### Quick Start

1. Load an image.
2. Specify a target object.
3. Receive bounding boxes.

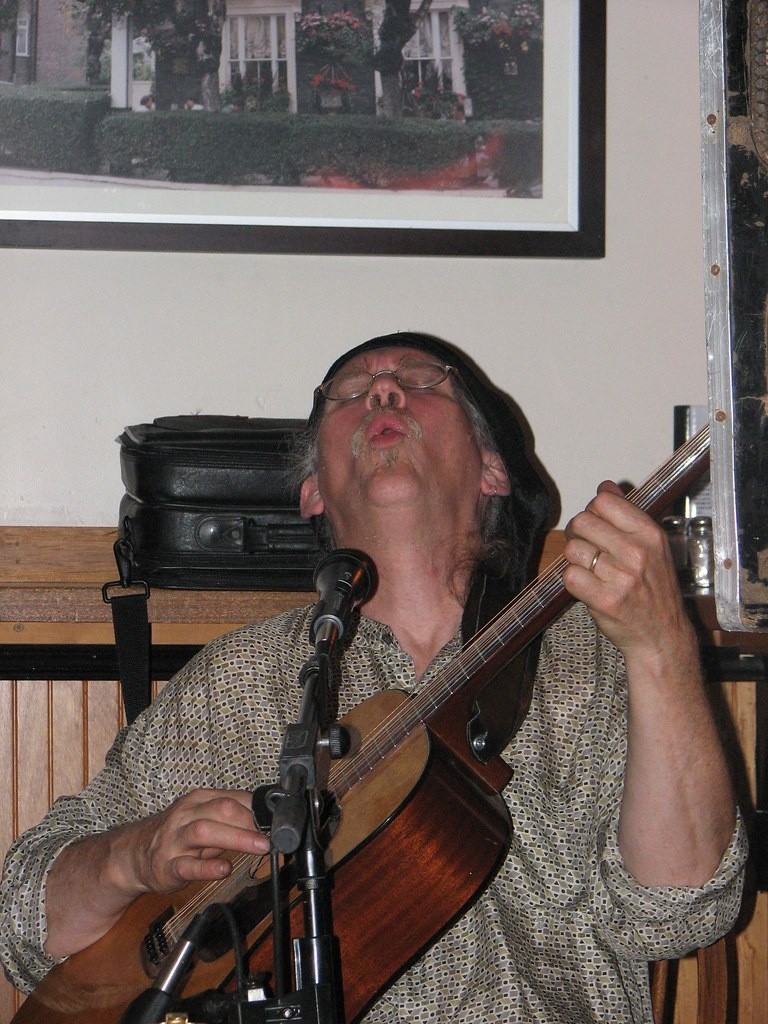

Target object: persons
[0,335,750,1024]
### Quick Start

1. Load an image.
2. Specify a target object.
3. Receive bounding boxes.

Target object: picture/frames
[1,0,608,261]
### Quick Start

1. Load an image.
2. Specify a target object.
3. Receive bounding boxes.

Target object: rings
[589,551,600,573]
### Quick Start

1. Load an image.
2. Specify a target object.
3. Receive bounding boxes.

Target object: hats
[306,332,548,594]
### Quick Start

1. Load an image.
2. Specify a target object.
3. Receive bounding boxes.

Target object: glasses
[311,362,486,459]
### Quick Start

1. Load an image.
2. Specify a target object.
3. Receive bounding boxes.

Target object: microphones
[312,547,379,663]
[119,912,211,1024]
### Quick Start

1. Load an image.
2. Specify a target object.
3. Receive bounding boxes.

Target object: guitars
[9,418,714,1024]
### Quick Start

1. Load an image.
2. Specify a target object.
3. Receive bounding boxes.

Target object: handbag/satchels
[102,415,313,725]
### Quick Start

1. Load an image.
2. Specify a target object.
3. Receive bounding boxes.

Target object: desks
[1,526,768,1024]
